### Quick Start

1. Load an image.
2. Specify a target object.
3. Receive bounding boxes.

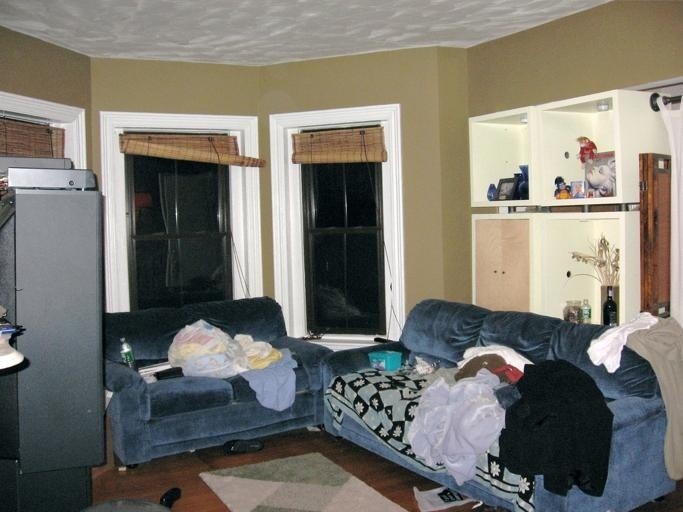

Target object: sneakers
[222,436,264,454]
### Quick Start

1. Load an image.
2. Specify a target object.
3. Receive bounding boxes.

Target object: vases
[601,286,617,326]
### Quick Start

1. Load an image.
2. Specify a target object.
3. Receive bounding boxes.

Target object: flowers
[569,234,619,287]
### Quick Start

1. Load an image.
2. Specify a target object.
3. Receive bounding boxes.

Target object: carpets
[198,452,409,512]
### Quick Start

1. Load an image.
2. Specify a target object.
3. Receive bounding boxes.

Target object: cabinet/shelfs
[474,218,529,314]
[532,215,623,326]
[468,91,621,204]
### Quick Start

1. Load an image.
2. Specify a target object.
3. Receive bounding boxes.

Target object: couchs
[326,298,678,510]
[101,298,332,469]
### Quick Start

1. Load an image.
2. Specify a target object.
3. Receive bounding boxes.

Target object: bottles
[604,286,617,327]
[120,337,136,371]
[563,299,591,325]
